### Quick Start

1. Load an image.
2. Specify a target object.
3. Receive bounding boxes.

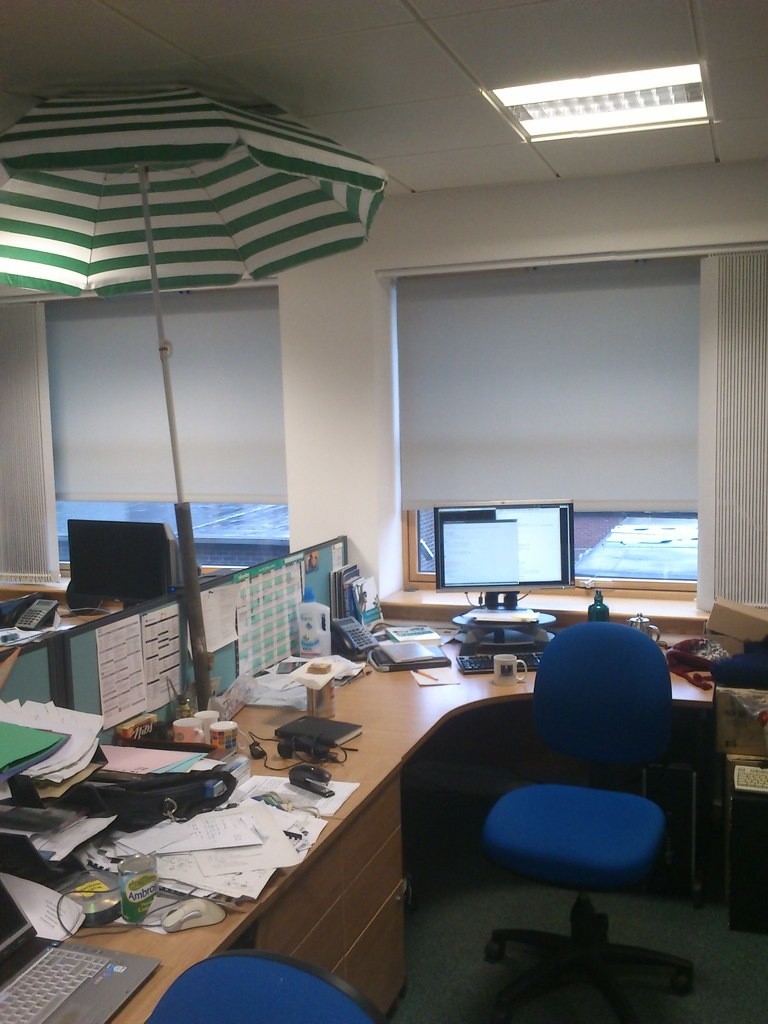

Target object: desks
[0,535,718,1024]
[724,754,768,932]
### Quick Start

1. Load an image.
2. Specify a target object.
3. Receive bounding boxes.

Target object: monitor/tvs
[67,518,183,602]
[433,499,576,594]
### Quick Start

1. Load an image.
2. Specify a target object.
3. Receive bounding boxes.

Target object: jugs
[627,613,661,643]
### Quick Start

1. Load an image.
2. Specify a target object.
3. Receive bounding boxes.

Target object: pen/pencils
[351,671,373,682]
[340,676,354,687]
[413,669,439,682]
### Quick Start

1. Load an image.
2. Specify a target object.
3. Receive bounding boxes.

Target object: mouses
[162,898,226,933]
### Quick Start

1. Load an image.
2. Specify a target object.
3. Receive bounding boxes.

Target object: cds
[53,870,121,927]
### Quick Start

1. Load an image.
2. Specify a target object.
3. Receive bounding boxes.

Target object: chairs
[481,622,695,1024]
[145,950,390,1024]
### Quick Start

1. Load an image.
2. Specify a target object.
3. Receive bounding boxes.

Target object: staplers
[288,765,336,798]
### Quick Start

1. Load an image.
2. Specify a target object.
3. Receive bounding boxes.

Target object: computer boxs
[642,764,696,895]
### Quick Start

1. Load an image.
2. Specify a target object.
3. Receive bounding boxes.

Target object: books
[330,564,383,631]
[385,625,441,646]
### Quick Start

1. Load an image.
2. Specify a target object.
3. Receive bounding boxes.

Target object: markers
[2,633,20,644]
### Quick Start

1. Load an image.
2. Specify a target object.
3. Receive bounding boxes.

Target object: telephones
[3,595,59,631]
[331,617,379,655]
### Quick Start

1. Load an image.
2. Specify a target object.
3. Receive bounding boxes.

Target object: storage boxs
[715,681,768,756]
[116,714,156,747]
[705,596,768,642]
[703,622,744,656]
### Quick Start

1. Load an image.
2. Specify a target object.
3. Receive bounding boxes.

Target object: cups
[209,720,250,755]
[193,710,220,745]
[172,716,207,744]
[493,654,528,686]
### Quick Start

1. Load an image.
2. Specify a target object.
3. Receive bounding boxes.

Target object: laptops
[0,880,162,1024]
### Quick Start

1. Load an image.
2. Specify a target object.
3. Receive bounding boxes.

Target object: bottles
[298,587,331,659]
[588,589,610,622]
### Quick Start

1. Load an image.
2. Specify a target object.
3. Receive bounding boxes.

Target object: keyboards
[456,652,542,675]
